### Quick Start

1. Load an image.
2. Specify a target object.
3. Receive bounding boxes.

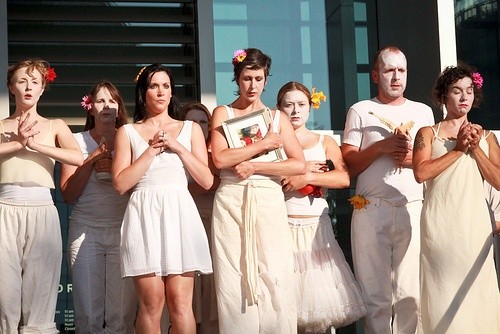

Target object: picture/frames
[221,107,284,163]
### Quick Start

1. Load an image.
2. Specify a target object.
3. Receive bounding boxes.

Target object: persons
[60,62,222,334]
[276,81,366,334]
[413,65,500,334]
[0,59,83,334]
[342,47,435,334]
[212,49,306,334]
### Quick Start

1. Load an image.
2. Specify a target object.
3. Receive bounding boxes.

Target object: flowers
[81,95,94,111]
[348,196,370,210]
[310,86,327,109]
[232,49,248,64]
[44,67,57,83]
[471,73,484,90]
